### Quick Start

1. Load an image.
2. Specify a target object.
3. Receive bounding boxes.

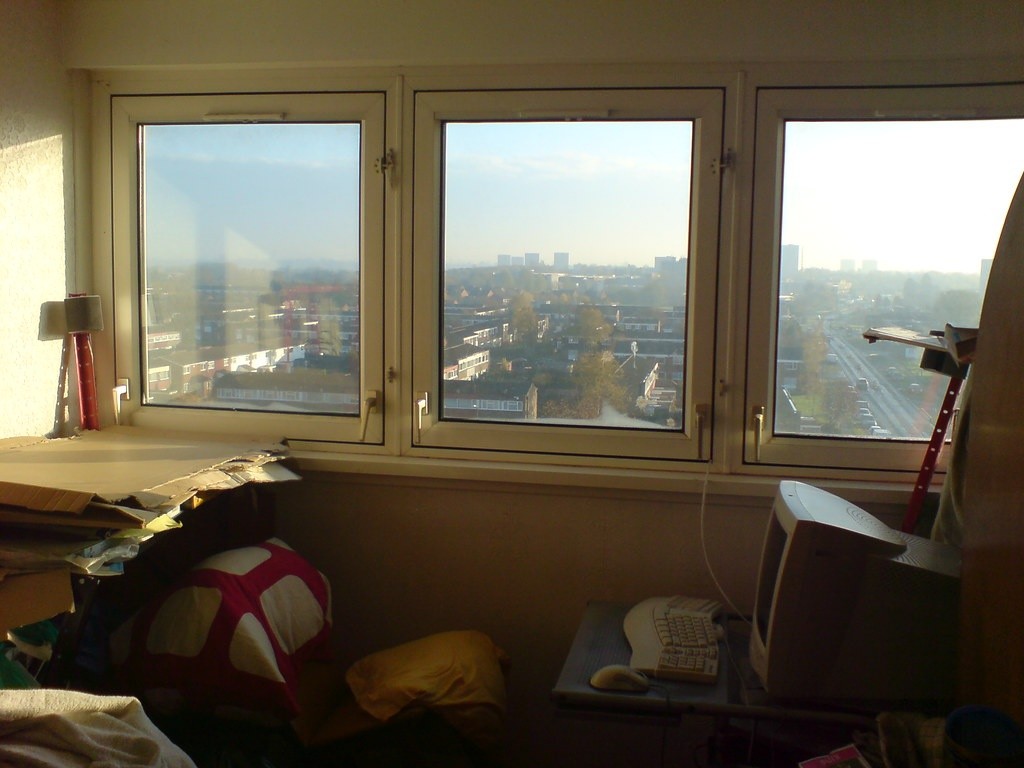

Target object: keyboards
[623,595,727,684]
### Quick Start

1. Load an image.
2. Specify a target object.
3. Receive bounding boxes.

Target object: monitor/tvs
[749,479,964,704]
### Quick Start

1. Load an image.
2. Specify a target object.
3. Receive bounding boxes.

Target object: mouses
[589,664,650,691]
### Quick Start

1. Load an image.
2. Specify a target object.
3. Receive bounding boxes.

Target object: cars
[855,400,869,409]
[868,425,881,434]
[861,413,875,420]
[873,429,890,438]
[860,407,871,414]
[856,378,869,390]
[863,420,877,427]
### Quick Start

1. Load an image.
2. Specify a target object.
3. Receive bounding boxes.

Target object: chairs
[201,537,510,768]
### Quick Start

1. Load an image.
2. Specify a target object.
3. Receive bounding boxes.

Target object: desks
[551,600,880,768]
